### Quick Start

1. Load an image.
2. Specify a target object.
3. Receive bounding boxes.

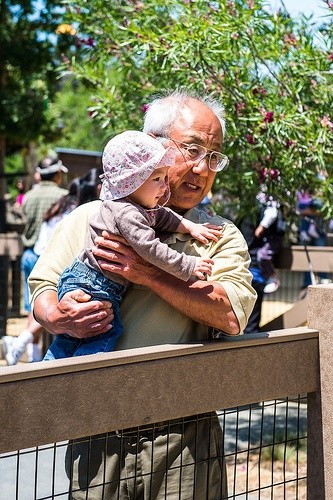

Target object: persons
[64,89,258,500]
[42,129,223,365]
[237,173,329,333]
[0,153,102,366]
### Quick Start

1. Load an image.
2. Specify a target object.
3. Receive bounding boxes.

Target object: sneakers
[0,336,24,366]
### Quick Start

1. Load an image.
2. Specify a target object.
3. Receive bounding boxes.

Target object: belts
[91,415,196,438]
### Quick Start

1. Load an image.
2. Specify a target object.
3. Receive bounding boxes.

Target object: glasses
[158,136,229,172]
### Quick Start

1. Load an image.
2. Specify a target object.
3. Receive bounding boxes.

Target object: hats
[35,160,68,174]
[100,130,171,200]
